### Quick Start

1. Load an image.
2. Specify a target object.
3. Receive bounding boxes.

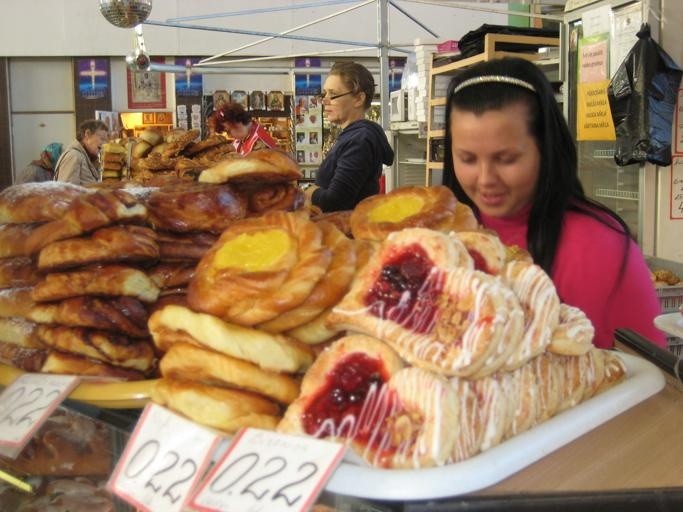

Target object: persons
[215,95,226,110]
[311,151,320,163]
[297,98,307,115]
[442,58,669,360]
[54,119,110,185]
[297,133,305,145]
[310,133,318,144]
[301,59,394,214]
[17,143,62,184]
[213,104,275,156]
[270,94,282,108]
[141,71,153,91]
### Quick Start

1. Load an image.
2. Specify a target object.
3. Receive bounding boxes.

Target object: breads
[135,130,240,188]
[149,184,478,433]
[649,269,683,288]
[280,228,623,468]
[2,150,304,382]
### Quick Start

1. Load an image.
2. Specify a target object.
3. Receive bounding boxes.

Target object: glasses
[317,85,378,106]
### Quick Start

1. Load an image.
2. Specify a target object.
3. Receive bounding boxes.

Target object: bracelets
[302,183,312,192]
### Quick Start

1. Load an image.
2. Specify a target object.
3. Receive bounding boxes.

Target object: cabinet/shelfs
[0,378,681,512]
[424,33,562,190]
[200,83,295,156]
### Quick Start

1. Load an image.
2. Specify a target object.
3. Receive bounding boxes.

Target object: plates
[652,308,682,341]
